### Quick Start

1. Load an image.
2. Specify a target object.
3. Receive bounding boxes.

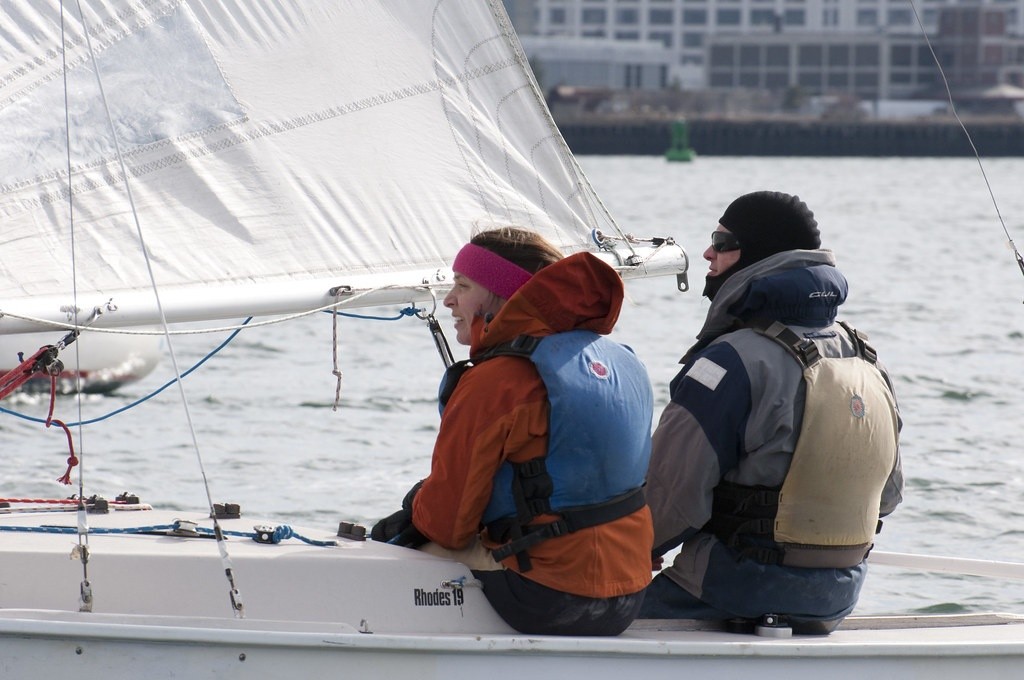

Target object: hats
[718,191,821,260]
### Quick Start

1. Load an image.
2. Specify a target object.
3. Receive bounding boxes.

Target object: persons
[636,190,903,637]
[372,227,654,637]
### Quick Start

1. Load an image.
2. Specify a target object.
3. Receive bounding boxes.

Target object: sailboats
[0,0,1024,679]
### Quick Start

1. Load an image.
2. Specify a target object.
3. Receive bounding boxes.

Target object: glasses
[711,231,739,253]
